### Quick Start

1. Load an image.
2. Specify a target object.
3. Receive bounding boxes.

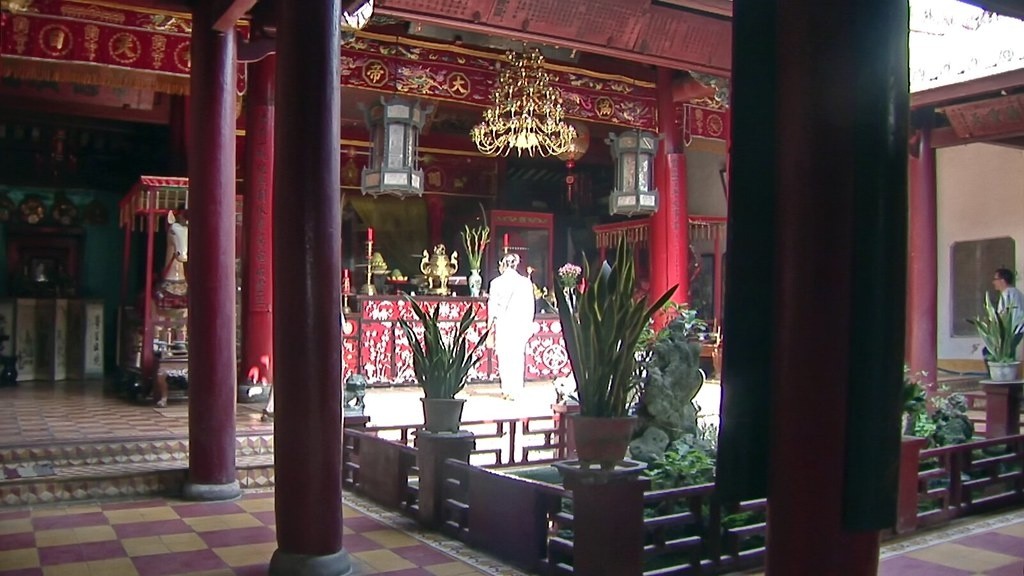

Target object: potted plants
[398,289,490,436]
[551,230,681,470]
[966,287,1024,382]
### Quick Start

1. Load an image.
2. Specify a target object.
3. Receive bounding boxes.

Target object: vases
[469,269,481,297]
[563,285,576,316]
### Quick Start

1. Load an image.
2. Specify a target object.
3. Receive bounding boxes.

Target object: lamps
[469,42,580,156]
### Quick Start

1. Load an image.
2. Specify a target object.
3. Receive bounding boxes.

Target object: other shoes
[500,393,515,400]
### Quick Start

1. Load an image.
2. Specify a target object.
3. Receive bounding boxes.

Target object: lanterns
[604,129,665,218]
[360,94,434,199]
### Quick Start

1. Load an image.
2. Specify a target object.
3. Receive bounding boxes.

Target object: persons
[992,269,1024,360]
[487,253,535,400]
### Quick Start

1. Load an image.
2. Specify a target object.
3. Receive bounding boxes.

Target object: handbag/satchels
[486,323,496,349]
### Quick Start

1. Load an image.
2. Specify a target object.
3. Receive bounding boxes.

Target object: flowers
[557,263,580,286]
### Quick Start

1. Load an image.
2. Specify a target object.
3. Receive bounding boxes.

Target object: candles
[369,227,372,241]
[345,269,348,279]
[504,233,508,247]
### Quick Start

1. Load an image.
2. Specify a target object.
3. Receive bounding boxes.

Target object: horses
[151,209,188,354]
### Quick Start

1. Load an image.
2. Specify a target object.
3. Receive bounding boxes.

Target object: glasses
[992,277,1002,281]
